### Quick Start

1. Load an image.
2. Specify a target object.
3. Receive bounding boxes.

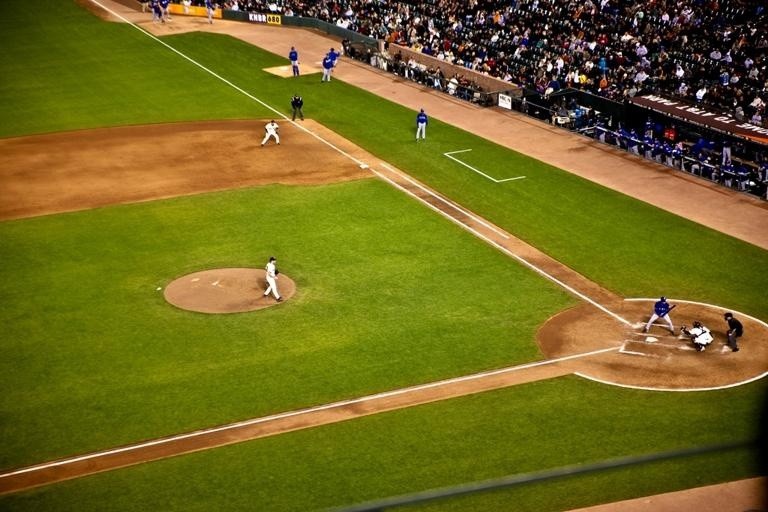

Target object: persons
[644,296,676,337]
[262,256,283,302]
[724,313,744,353]
[416,109,428,141]
[150,0,319,18]
[289,47,300,76]
[290,94,304,120]
[319,0,526,112]
[680,319,714,352]
[261,120,280,146]
[208,6,213,22]
[152,3,170,21]
[526,0,768,199]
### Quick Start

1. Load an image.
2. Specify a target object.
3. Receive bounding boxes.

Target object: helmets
[692,321,700,328]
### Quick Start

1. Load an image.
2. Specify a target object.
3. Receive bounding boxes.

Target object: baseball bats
[662,305,675,317]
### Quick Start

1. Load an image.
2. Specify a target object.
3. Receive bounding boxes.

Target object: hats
[724,313,732,317]
[421,109,424,112]
[661,297,666,302]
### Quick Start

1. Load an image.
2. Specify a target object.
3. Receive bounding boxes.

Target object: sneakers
[732,347,737,352]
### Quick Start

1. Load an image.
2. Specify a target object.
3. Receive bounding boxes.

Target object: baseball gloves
[680,326,686,333]
